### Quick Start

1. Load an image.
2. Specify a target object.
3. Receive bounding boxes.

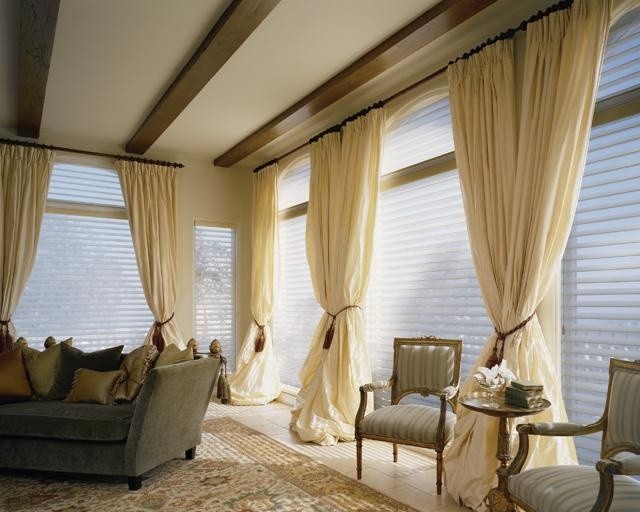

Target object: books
[504,380,543,409]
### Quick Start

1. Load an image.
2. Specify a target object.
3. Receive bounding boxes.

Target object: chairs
[502,358,640,512]
[353,335,463,495]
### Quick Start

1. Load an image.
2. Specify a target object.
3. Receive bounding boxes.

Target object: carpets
[1,416,423,512]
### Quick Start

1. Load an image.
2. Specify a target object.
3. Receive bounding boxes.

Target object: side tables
[456,390,552,512]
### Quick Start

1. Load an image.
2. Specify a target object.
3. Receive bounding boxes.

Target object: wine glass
[477,377,506,410]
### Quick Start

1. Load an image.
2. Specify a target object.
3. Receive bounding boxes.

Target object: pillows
[0,336,195,407]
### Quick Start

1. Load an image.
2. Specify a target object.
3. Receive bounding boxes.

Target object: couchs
[0,335,222,490]
